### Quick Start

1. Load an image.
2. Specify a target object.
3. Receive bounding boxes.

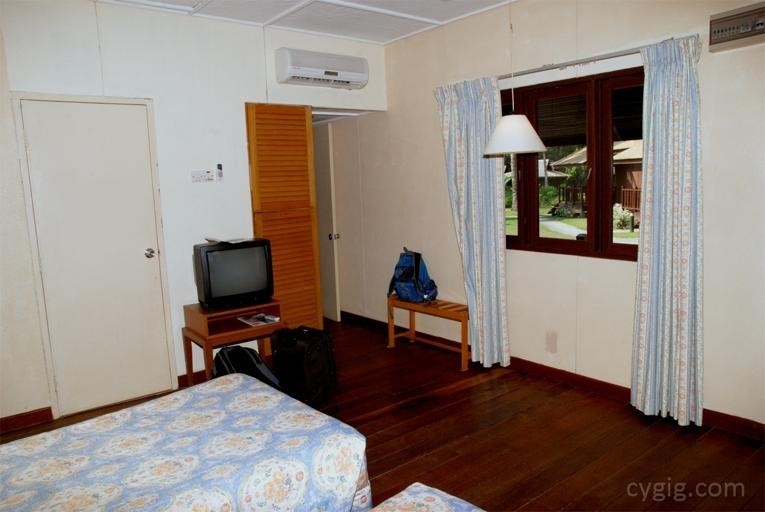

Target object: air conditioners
[273,45,373,91]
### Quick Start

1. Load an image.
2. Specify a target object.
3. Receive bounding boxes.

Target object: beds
[0,373,487,510]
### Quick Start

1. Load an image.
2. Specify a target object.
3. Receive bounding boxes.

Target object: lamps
[480,0,551,159]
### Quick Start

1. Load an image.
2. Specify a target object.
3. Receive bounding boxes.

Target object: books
[237,314,281,328]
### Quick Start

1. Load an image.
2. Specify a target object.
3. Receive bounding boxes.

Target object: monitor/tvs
[193,237,274,312]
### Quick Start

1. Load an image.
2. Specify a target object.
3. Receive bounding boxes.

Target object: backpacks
[387,247,437,303]
[211,346,284,392]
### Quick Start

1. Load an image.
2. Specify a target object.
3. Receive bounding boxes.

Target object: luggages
[271,325,336,404]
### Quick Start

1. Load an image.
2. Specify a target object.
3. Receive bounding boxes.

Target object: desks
[387,292,483,372]
[181,300,289,385]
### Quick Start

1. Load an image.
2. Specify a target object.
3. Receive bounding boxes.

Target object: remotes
[266,315,280,322]
